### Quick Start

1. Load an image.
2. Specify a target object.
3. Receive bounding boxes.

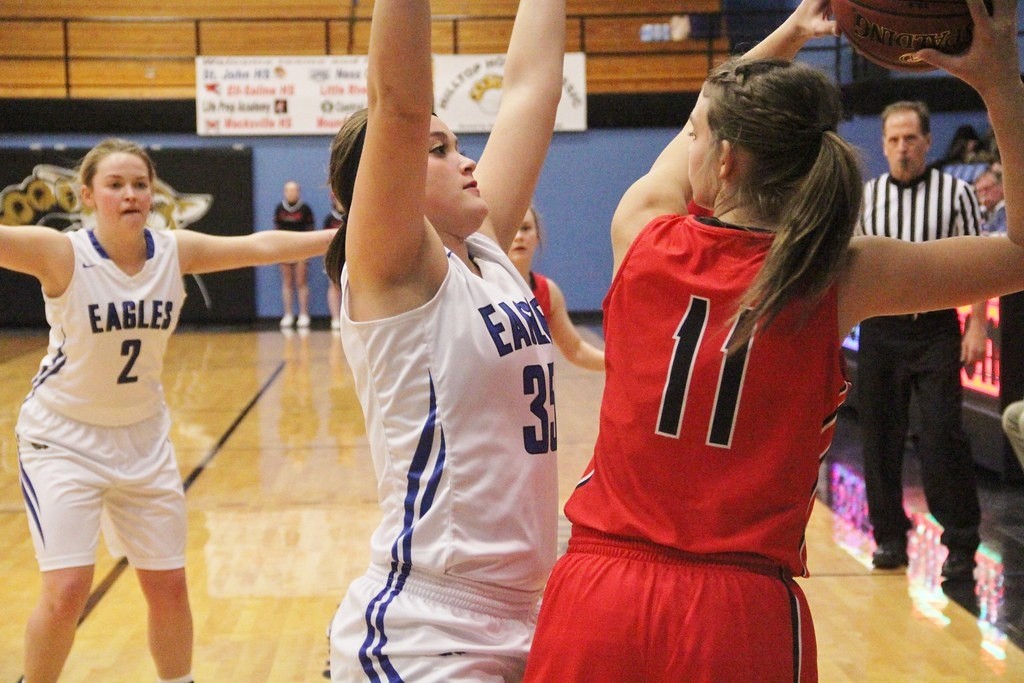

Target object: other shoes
[941,553,977,577]
[279,315,295,327]
[296,315,310,327]
[331,318,340,330]
[872,537,908,567]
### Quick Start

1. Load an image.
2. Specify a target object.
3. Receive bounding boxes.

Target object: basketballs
[830,0,993,73]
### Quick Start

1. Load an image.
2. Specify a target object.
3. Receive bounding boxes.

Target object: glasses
[973,181,1000,196]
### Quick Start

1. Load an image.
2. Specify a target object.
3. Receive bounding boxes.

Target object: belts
[884,309,952,324]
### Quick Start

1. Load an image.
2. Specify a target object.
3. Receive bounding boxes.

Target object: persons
[852,89,1024,584]
[520,0,1024,683]
[0,140,337,683]
[270,0,606,683]
